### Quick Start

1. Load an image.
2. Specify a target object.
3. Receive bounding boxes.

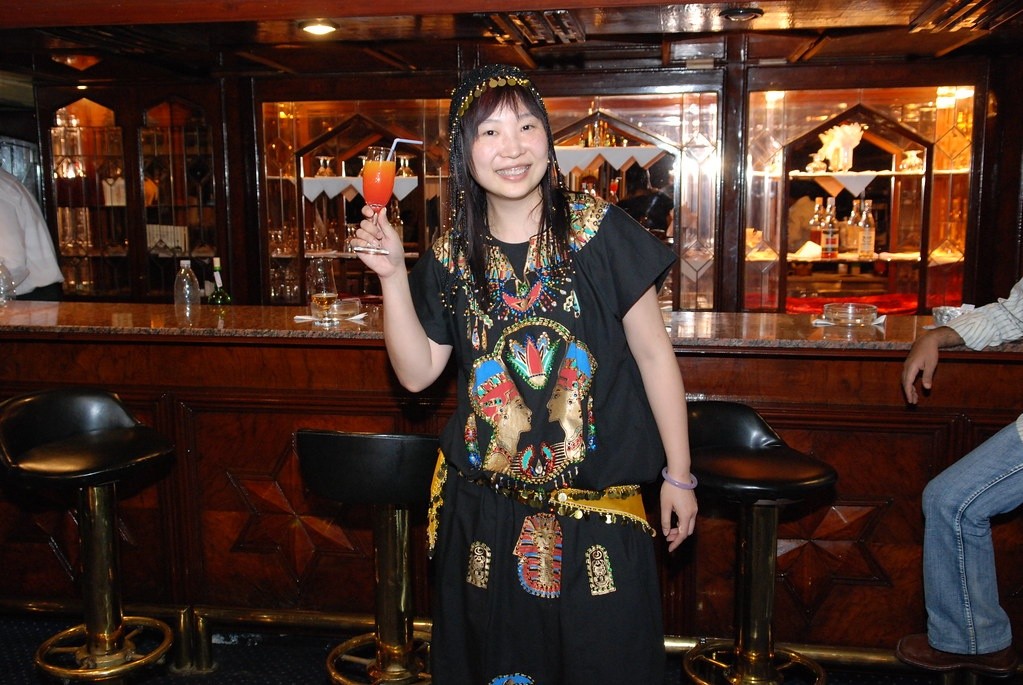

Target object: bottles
[579,105,616,147]
[809,196,825,246]
[208,257,231,306]
[846,199,861,253]
[821,198,839,257]
[390,199,404,246]
[858,200,875,257]
[174,260,201,325]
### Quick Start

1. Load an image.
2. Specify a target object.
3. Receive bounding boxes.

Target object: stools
[686,400,839,685]
[294,428,443,685]
[0,386,179,681]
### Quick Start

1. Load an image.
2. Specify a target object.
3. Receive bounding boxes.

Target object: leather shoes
[897,633,1014,677]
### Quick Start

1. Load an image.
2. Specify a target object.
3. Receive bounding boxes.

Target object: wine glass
[316,154,415,177]
[310,258,338,327]
[901,150,923,171]
[269,222,360,254]
[352,144,396,254]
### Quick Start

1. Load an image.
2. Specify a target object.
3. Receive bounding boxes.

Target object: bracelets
[661,467,697,490]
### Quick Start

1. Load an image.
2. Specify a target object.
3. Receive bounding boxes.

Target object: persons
[353,71,699,685]
[612,163,676,297]
[894,277,1023,676]
[1,166,68,301]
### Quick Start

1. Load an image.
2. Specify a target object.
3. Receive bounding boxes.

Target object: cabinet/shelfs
[43,122,962,318]
